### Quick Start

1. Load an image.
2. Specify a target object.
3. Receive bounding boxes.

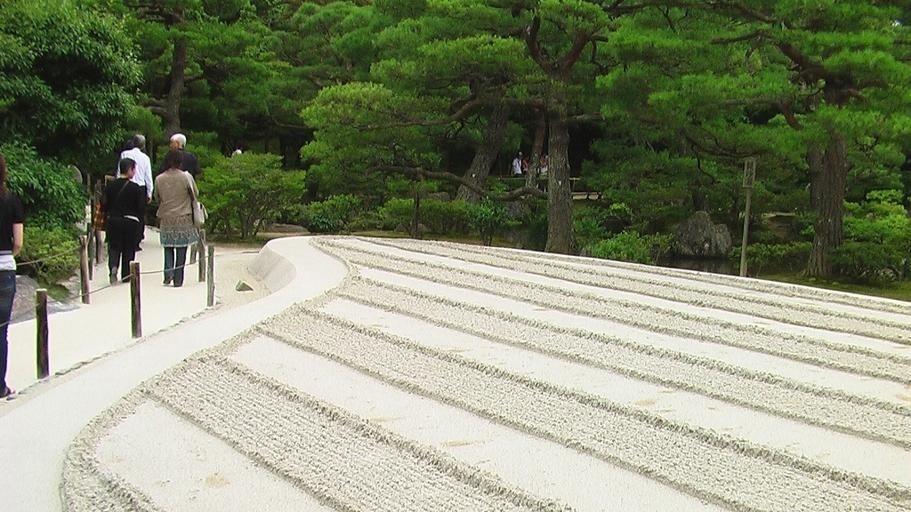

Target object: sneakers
[109,263,186,287]
[1,385,16,398]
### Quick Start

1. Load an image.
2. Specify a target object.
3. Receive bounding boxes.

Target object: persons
[0,152,27,399]
[115,133,156,253]
[509,150,549,176]
[232,142,244,156]
[158,132,199,182]
[154,151,200,289]
[98,156,148,285]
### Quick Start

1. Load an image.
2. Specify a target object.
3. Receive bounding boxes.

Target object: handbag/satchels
[185,168,209,225]
